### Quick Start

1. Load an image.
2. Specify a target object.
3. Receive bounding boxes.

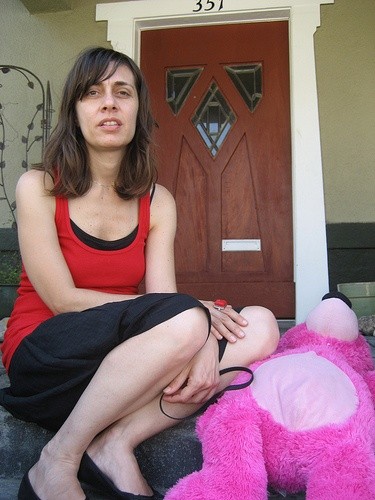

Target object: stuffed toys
[164,293,375,500]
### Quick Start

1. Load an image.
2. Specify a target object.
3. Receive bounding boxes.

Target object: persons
[0,45,279,500]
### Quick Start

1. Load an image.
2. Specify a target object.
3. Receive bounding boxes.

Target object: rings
[213,299,228,311]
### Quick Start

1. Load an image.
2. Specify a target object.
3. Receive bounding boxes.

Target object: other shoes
[18,467,89,500]
[78,450,165,500]
